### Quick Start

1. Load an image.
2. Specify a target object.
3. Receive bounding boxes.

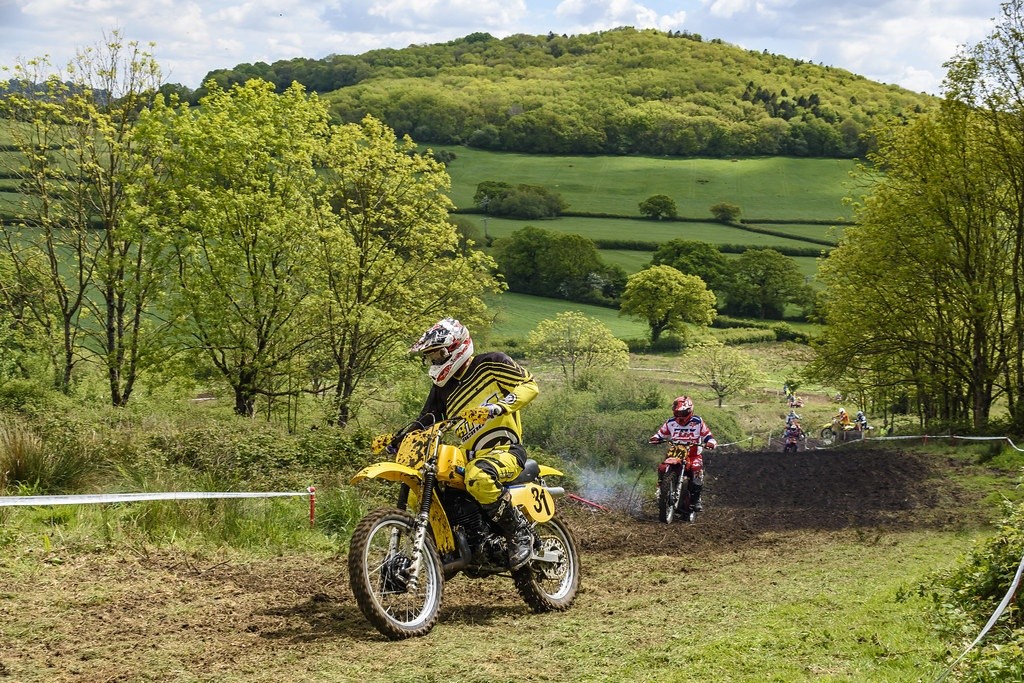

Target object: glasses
[422,325,469,367]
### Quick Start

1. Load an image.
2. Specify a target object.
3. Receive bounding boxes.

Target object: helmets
[671,396,694,426]
[790,410,795,416]
[856,411,864,418]
[790,424,798,433]
[409,316,474,387]
[839,408,846,415]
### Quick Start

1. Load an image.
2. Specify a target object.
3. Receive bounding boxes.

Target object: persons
[782,410,805,445]
[650,395,717,513]
[384,315,542,571]
[835,408,851,431]
[854,411,867,430]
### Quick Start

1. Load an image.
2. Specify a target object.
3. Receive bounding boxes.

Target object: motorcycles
[657,438,712,524]
[784,417,874,457]
[347,405,582,640]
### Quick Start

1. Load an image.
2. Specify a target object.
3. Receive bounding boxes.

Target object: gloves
[480,402,506,418]
[650,438,659,444]
[385,436,400,455]
[705,442,714,450]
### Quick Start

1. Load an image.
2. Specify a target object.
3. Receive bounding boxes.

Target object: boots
[690,482,702,510]
[488,495,535,571]
